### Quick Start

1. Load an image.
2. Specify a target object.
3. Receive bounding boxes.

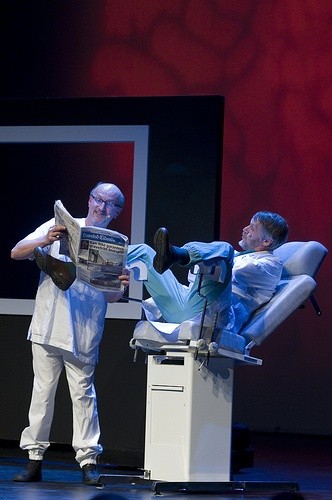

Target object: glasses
[92,195,120,208]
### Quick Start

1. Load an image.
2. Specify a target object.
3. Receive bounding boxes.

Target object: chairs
[124,242,328,366]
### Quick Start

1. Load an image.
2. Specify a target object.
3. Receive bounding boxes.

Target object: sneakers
[13,461,42,481]
[82,463,99,484]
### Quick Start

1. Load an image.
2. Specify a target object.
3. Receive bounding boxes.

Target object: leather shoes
[33,247,76,291]
[153,227,173,274]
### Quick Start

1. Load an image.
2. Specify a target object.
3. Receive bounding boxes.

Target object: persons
[33,211,289,335]
[11,183,130,487]
